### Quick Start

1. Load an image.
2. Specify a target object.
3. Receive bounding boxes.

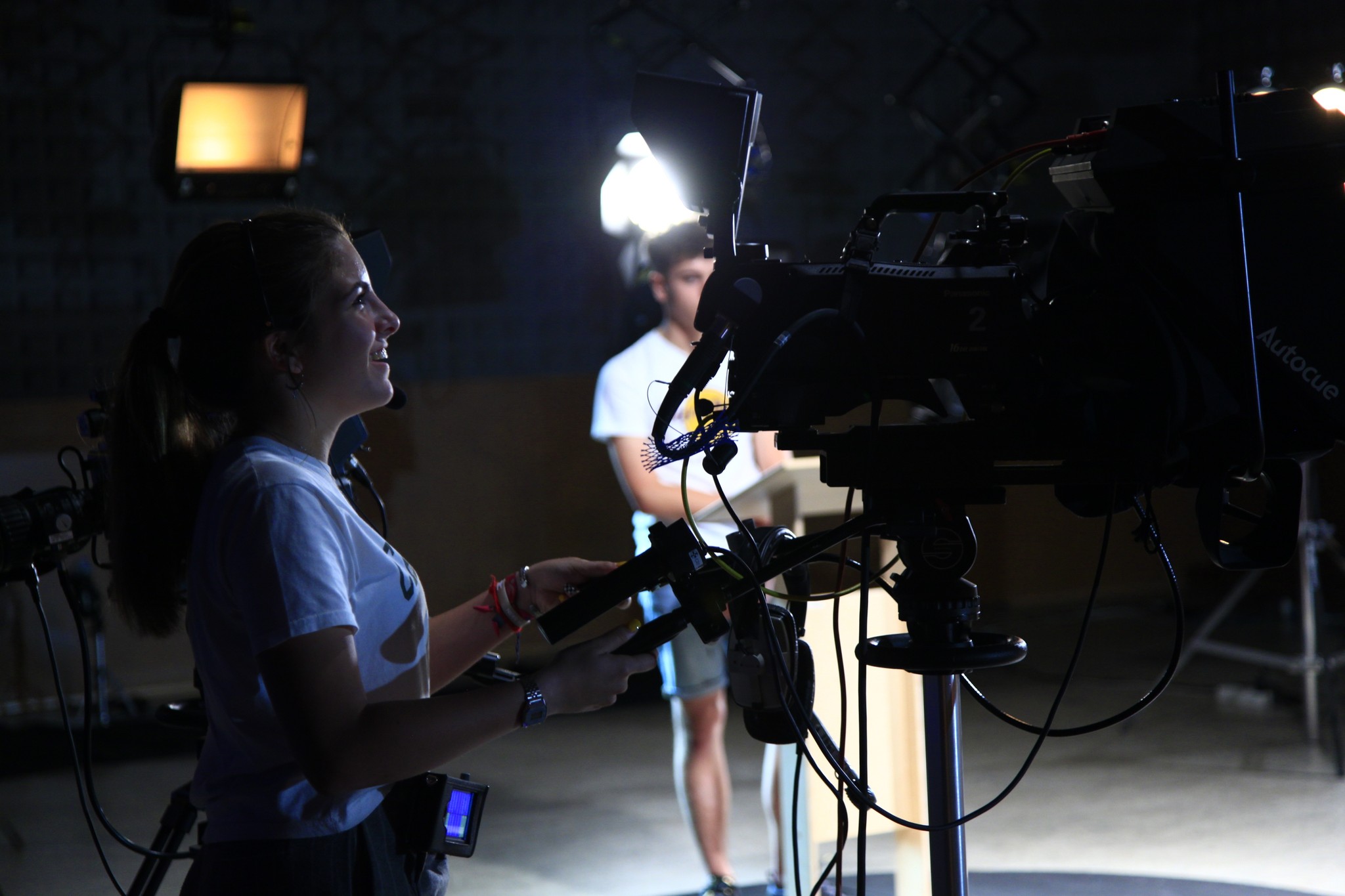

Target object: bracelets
[472,565,543,638]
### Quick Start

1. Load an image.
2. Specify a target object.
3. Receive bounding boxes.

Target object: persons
[96,208,659,896]
[591,222,811,894]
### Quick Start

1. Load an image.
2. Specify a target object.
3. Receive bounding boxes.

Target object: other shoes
[701,877,736,896]
[766,878,846,896]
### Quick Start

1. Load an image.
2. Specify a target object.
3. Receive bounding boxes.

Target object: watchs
[516,675,547,727]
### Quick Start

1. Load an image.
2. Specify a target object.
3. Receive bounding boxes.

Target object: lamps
[164,67,315,207]
